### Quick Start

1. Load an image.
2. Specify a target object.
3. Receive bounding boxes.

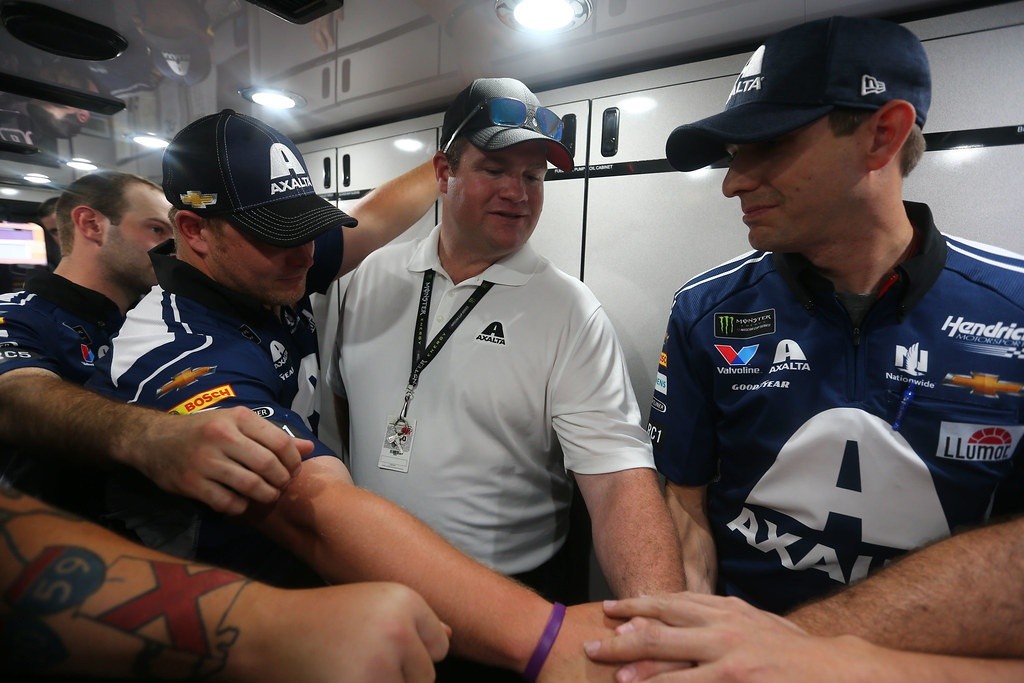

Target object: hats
[162,108,359,249]
[664,16,933,173]
[440,77,574,173]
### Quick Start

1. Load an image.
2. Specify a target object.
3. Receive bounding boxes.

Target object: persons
[314,77,687,600]
[583,590,1024,682]
[110,107,634,683]
[1,0,345,140]
[0,172,317,525]
[645,15,1023,656]
[38,198,63,245]
[0,480,455,683]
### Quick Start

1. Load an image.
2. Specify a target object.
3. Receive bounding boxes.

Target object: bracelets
[521,601,567,683]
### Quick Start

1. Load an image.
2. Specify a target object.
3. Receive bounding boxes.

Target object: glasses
[442,97,563,153]
